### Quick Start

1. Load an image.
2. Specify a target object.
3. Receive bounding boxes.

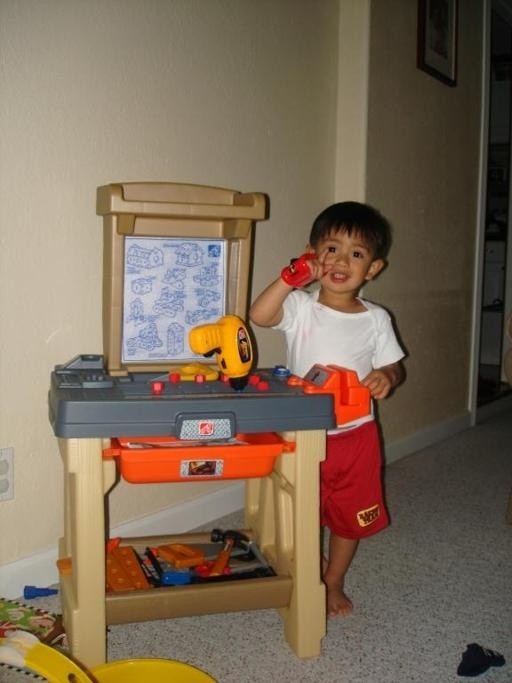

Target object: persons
[247,201,408,618]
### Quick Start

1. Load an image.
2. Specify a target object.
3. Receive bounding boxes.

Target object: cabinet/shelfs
[479,241,505,386]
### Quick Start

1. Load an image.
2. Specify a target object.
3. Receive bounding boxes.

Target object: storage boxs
[113,433,295,484]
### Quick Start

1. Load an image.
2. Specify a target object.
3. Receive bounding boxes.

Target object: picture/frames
[417,0,458,86]
